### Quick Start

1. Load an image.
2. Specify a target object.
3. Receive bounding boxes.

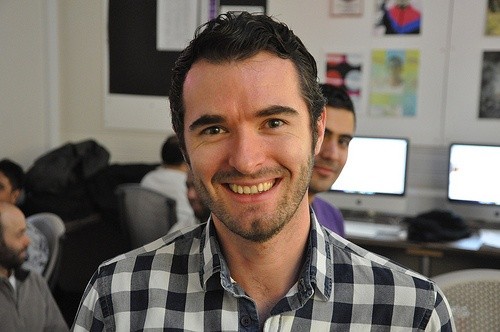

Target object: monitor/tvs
[326,136,409,196]
[446,142,500,207]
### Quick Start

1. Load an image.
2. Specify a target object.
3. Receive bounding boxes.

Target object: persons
[67,11,456,332]
[0,201,69,331]
[137,133,199,235]
[0,159,25,205]
[307,81,356,238]
